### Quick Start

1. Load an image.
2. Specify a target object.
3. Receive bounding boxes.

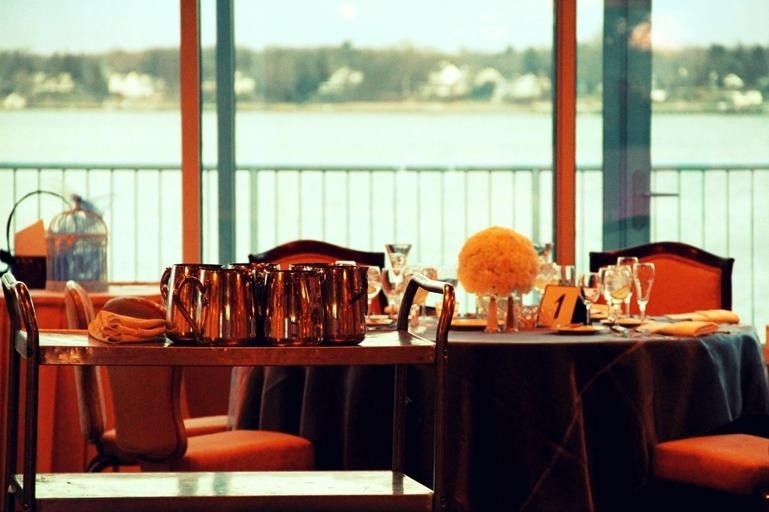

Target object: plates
[363,314,400,325]
[448,318,505,328]
[552,323,604,334]
[598,319,653,326]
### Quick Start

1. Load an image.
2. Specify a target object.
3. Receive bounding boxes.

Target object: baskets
[6,191,78,289]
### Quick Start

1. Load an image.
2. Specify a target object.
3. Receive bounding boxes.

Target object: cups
[159,262,368,347]
[369,266,382,304]
[385,242,411,276]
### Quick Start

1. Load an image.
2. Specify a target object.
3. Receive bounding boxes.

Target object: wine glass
[579,256,655,325]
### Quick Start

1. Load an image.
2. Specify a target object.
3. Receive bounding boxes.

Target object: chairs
[586,240,736,314]
[248,241,395,311]
[89,295,313,476]
[57,280,231,468]
[656,432,769,507]
